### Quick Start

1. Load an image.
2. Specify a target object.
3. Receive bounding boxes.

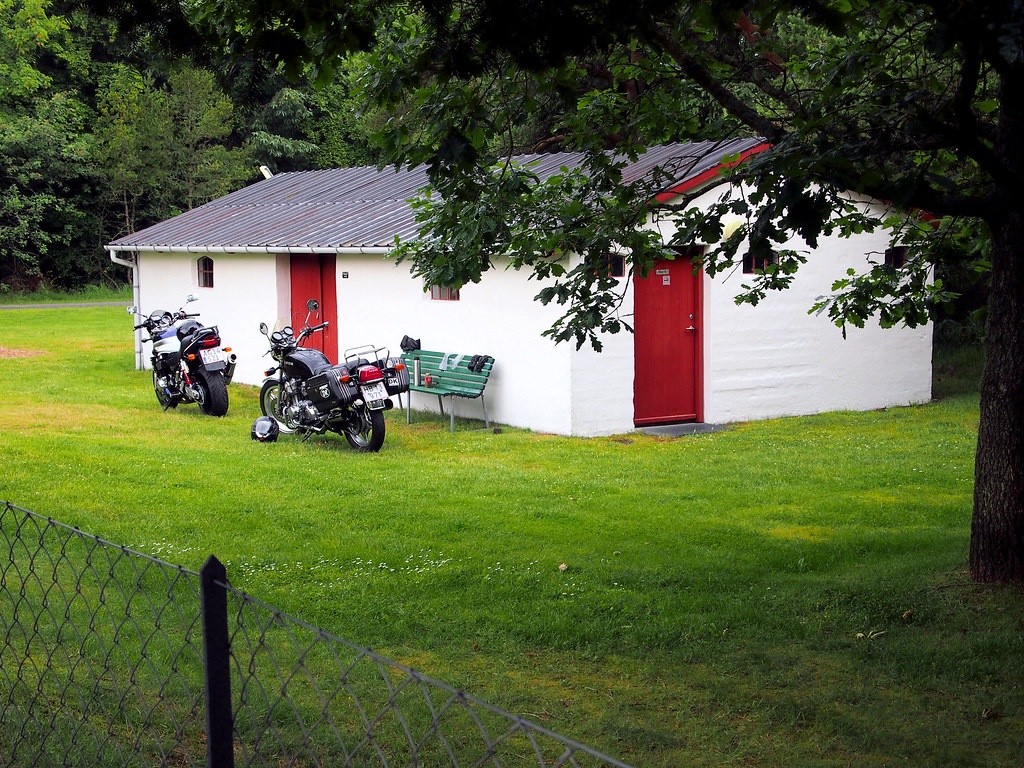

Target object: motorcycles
[127,294,238,418]
[260,299,411,454]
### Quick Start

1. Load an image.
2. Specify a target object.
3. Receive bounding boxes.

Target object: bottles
[425,373,432,387]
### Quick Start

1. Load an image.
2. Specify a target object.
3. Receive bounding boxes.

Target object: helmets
[176,319,204,342]
[251,415,279,443]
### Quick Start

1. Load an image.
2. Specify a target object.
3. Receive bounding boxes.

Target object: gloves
[399,335,420,351]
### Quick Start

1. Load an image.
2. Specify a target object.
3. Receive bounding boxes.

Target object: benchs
[398,349,495,432]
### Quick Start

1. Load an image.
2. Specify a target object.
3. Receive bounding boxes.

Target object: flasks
[414,357,421,386]
